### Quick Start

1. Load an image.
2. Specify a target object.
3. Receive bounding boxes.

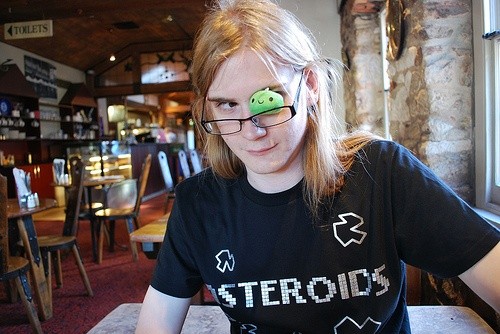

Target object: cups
[31,119,39,127]
[12,110,20,117]
[7,119,14,126]
[17,119,25,127]
[0,118,7,126]
[30,111,35,118]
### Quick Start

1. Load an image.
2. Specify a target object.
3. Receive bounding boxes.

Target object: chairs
[0,149,202,334]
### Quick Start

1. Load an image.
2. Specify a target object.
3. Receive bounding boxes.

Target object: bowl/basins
[1,128,26,139]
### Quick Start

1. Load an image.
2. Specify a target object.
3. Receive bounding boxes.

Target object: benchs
[88,301,499,334]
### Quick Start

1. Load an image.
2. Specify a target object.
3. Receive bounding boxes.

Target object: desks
[51,175,125,262]
[8,199,58,320]
[130,213,170,242]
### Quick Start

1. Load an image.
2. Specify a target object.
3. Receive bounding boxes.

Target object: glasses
[201,67,305,136]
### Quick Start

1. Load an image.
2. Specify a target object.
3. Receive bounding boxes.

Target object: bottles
[57,174,68,185]
[27,192,39,209]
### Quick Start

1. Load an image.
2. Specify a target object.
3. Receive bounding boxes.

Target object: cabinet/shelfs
[0,64,99,140]
[67,142,185,210]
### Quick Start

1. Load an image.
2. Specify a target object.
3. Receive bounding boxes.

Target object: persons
[134,0,500,334]
